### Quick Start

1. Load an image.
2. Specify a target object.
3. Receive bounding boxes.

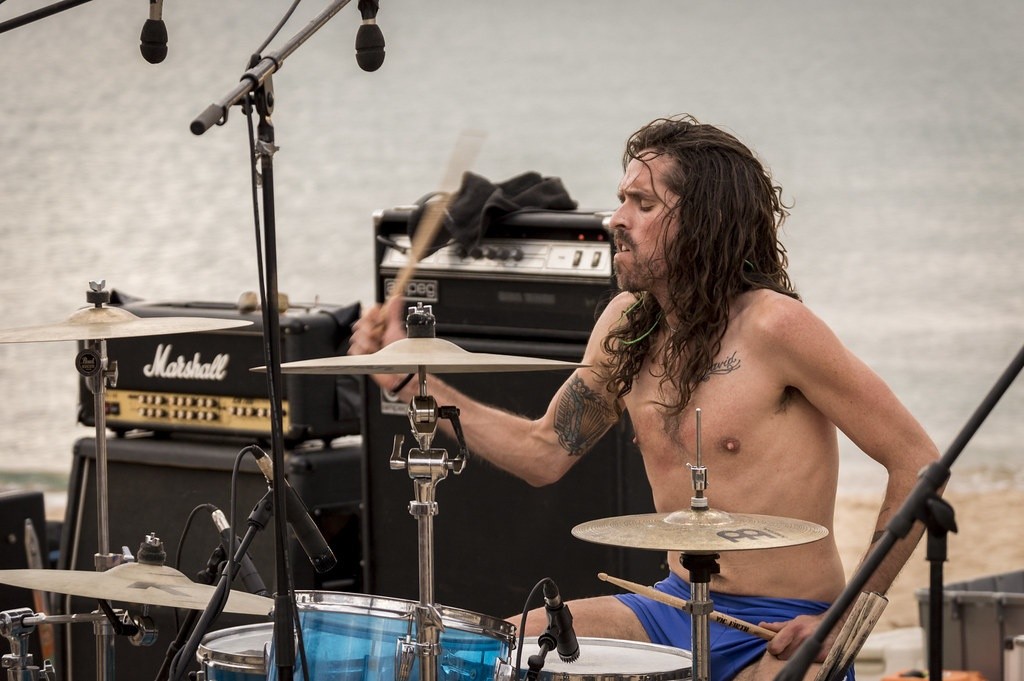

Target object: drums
[438,636,692,681]
[196,622,297,681]
[266,590,518,681]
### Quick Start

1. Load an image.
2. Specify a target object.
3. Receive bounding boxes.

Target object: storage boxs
[914,569,1024,681]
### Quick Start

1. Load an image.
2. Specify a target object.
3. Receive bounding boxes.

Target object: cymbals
[0,562,275,616]
[0,306,254,344]
[249,338,594,374]
[570,508,829,556]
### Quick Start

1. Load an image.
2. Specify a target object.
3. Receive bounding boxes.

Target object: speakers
[50,435,365,681]
[358,340,668,624]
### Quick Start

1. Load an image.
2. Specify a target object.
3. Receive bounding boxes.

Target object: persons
[347,114,950,681]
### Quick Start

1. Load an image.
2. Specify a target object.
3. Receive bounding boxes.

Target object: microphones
[139,0,167,63]
[249,445,338,573]
[541,578,580,662]
[354,0,386,72]
[204,502,267,596]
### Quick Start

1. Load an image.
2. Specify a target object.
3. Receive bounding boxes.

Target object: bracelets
[387,372,417,397]
